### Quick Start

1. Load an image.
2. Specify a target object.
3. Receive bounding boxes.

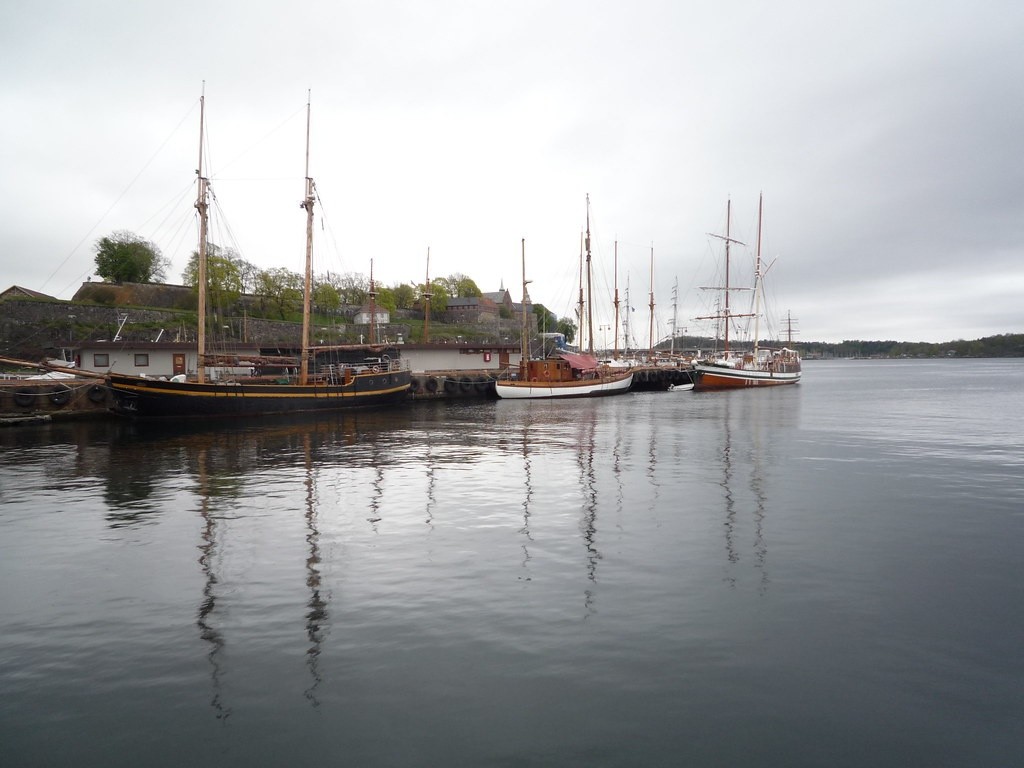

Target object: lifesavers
[12,388,37,406]
[459,378,472,392]
[425,378,437,392]
[444,378,457,393]
[408,377,420,391]
[474,377,488,393]
[87,384,108,403]
[48,384,71,405]
[489,376,499,391]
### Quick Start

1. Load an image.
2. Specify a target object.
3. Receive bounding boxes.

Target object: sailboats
[692,187,802,391]
[597,236,718,391]
[0,78,413,420]
[494,192,634,399]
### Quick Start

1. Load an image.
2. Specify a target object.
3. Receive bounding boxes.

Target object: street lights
[598,325,611,360]
